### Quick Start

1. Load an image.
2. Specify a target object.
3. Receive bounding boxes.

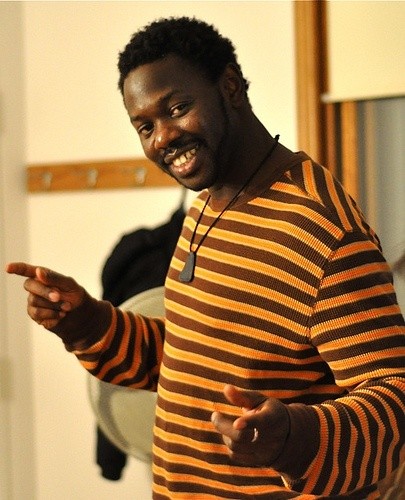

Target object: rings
[251,427,258,442]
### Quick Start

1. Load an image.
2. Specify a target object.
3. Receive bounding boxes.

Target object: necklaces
[178,135,280,282]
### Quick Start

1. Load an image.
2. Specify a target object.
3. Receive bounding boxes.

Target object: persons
[6,18,405,500]
